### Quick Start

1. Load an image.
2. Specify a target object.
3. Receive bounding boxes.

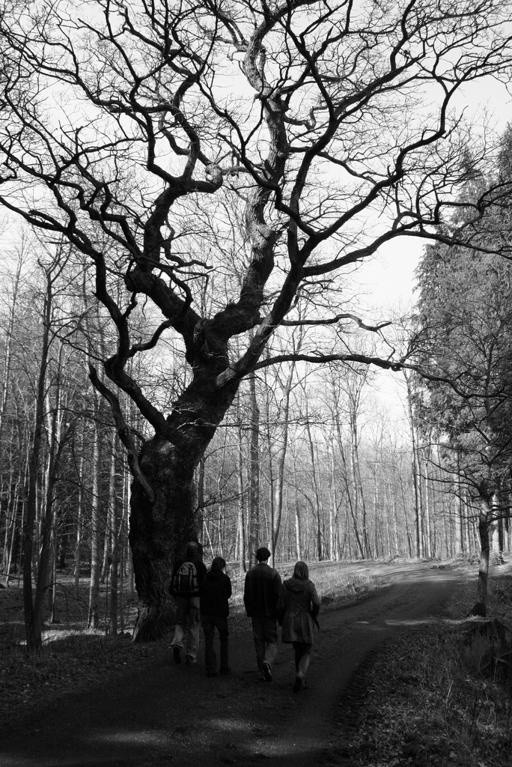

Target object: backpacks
[171,557,200,594]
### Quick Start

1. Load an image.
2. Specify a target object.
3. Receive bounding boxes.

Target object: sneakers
[295,675,305,691]
[206,665,231,677]
[172,645,182,665]
[185,654,194,666]
[262,663,272,682]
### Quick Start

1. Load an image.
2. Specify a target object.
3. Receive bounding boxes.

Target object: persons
[242,546,283,680]
[169,540,207,666]
[198,553,233,675]
[278,559,323,693]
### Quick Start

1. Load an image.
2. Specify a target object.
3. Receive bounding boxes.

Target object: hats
[256,547,271,560]
